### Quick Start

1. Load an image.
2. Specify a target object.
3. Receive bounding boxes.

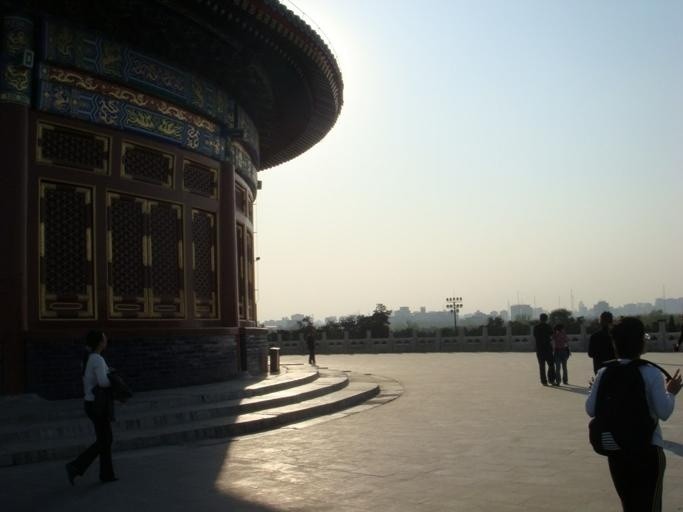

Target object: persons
[551,321,570,385]
[61,327,127,483]
[583,315,681,512]
[303,330,317,365]
[587,310,617,375]
[533,313,558,386]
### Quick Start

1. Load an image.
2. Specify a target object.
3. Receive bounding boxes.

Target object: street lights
[445,296,463,335]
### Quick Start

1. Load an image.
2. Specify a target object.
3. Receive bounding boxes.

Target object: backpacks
[588,360,671,456]
